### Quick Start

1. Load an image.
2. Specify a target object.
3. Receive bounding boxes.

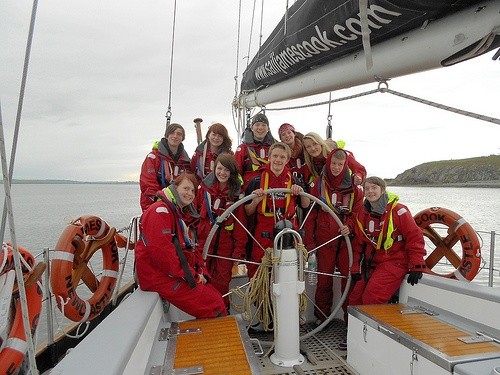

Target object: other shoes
[338,340,348,350]
[313,319,324,333]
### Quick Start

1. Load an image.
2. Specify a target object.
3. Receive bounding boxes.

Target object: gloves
[233,241,246,266]
[352,273,362,281]
[407,271,422,287]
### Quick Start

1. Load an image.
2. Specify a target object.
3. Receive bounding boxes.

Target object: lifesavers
[413,207,481,282]
[50,215,120,323]
[0,243,44,375]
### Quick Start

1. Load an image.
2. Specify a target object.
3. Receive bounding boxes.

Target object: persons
[279,123,306,168]
[138,123,195,216]
[131,173,225,318]
[314,147,366,326]
[296,132,367,186]
[242,140,313,274]
[188,124,234,178]
[190,153,243,313]
[237,114,283,181]
[338,176,427,348]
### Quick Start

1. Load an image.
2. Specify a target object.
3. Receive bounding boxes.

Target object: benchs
[346,303,500,375]
[152,315,263,375]
